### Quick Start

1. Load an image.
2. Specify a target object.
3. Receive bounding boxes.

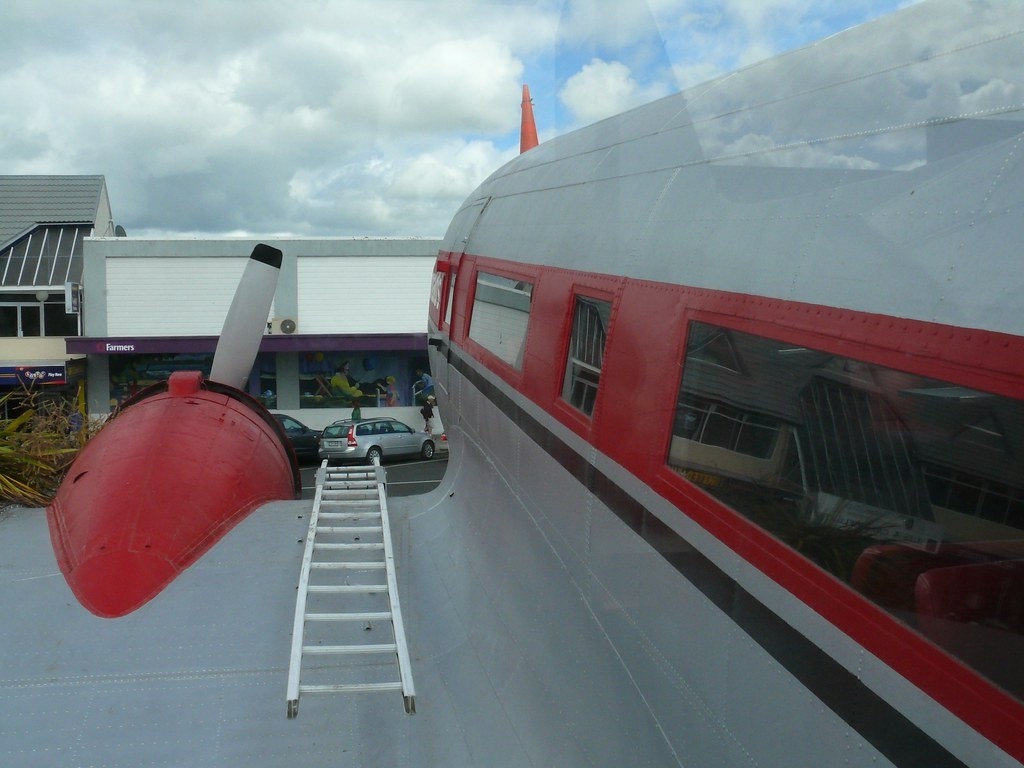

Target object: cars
[270,414,335,463]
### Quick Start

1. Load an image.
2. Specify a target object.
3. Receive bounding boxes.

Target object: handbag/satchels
[420,408,424,414]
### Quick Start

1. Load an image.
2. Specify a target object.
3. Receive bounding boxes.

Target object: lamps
[35,291,48,302]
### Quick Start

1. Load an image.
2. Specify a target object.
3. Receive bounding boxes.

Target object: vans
[668,353,946,567]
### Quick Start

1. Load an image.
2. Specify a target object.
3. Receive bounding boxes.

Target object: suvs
[316,416,437,461]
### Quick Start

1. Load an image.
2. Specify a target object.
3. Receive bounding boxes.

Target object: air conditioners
[272,317,298,335]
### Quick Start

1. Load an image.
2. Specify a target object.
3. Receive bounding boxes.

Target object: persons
[420,395,436,435]
[377,376,397,407]
[331,368,364,397]
[351,401,361,420]
[342,361,367,394]
[416,369,434,388]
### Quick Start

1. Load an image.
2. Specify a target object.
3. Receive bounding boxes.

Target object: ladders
[285,457,417,720]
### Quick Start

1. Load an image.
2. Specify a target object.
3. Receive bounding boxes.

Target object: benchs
[850,539,1023,689]
[325,428,348,437]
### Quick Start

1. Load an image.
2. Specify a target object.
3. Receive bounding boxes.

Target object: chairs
[362,426,368,434]
[379,426,386,433]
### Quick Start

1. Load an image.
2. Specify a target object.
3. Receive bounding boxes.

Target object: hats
[428,396,435,400]
